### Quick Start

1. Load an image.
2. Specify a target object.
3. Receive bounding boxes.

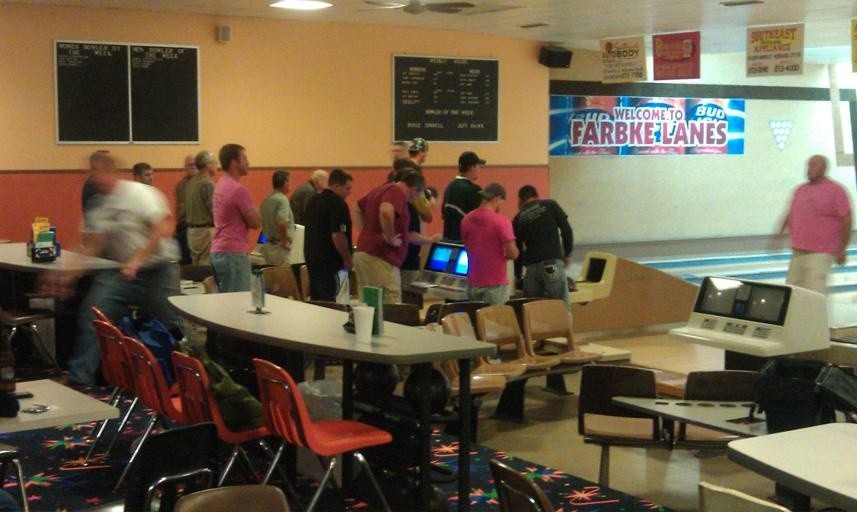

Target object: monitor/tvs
[256,230,267,245]
[423,242,455,273]
[691,275,744,317]
[743,283,794,326]
[453,248,470,276]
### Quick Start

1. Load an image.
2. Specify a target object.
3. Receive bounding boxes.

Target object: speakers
[538,46,574,68]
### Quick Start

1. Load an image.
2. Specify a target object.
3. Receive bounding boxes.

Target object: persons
[460,181,519,305]
[511,185,574,310]
[55,151,178,388]
[132,162,153,185]
[441,152,486,244]
[387,141,433,271]
[769,155,853,291]
[81,150,115,217]
[301,168,354,302]
[185,150,217,265]
[289,169,328,224]
[210,143,261,294]
[352,168,425,381]
[390,140,408,159]
[260,170,294,266]
[173,155,198,265]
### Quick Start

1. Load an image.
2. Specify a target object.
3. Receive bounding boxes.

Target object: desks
[725,422,852,512]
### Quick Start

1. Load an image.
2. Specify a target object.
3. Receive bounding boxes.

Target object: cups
[353,306,375,342]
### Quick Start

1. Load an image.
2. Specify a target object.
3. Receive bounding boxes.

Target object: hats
[458,151,485,171]
[410,137,427,152]
[479,183,504,199]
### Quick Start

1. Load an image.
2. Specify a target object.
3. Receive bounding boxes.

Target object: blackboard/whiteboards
[53,39,201,144]
[393,55,500,143]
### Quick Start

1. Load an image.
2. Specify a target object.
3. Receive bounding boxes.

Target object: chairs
[1,264,790,512]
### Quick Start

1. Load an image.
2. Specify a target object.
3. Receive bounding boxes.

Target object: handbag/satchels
[122,314,180,387]
[182,342,262,430]
